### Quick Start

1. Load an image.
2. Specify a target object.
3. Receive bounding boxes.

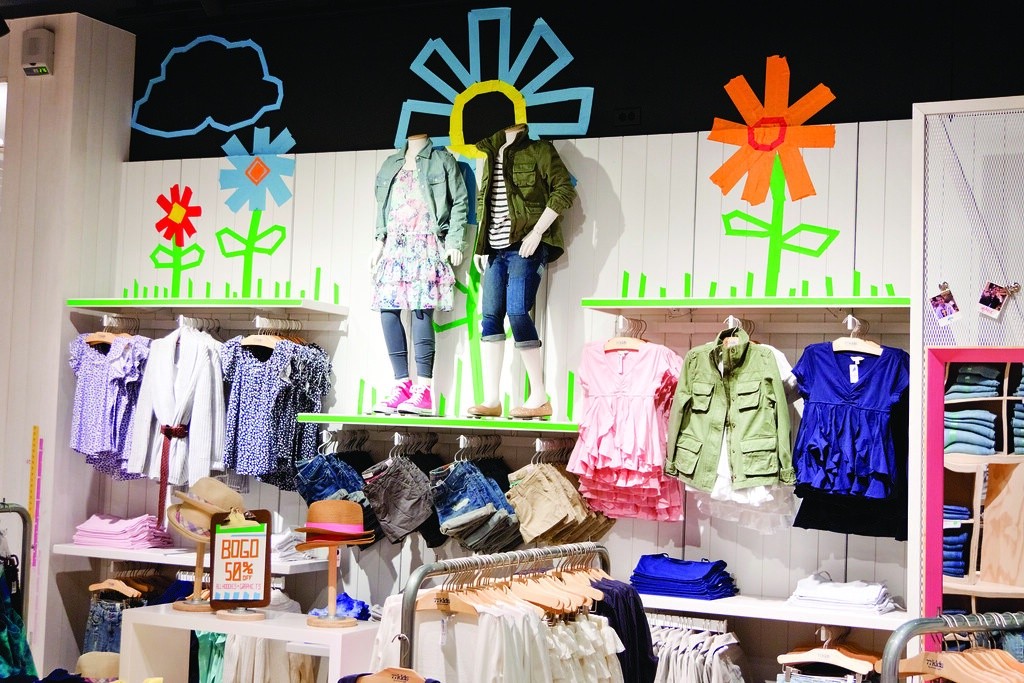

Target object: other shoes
[469,404,502,417]
[510,399,553,416]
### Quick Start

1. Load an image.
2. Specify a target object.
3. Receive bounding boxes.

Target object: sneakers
[397,384,432,415]
[375,380,413,413]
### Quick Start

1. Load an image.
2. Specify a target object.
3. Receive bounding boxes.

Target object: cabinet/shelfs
[48,93,1024,683]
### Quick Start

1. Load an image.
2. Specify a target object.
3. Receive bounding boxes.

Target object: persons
[373,132,469,414]
[931,298,937,309]
[979,286,1002,311]
[935,295,954,319]
[467,122,573,418]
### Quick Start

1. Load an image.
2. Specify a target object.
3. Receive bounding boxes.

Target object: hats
[167,478,245,544]
[293,500,375,551]
